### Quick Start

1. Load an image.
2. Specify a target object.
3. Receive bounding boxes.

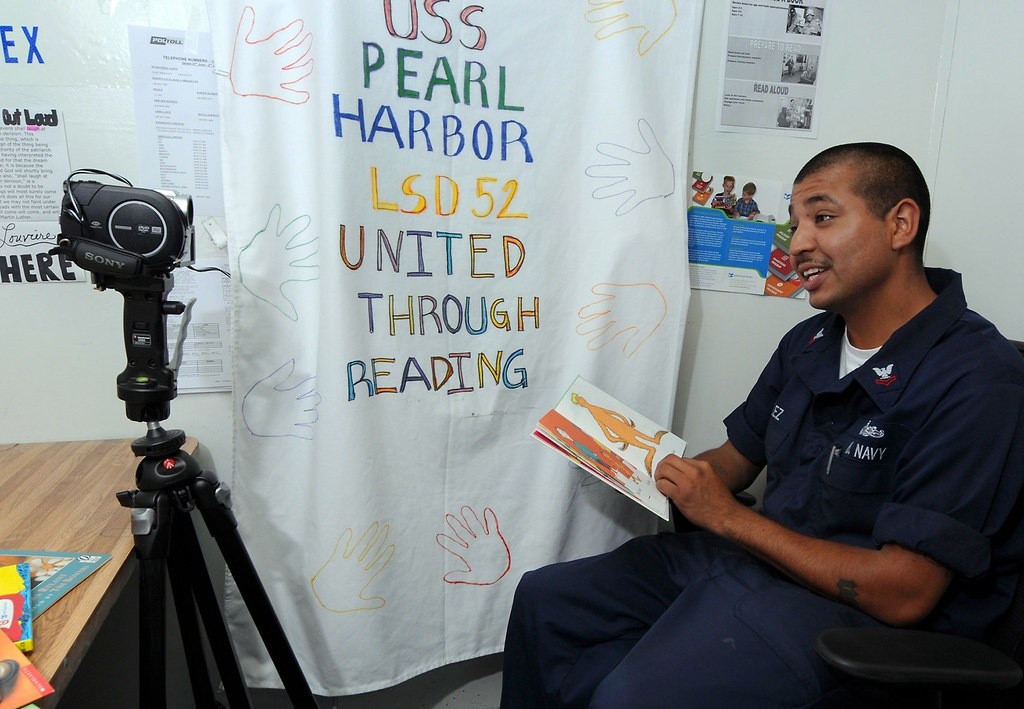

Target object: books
[0,549,113,709]
[530,374,689,525]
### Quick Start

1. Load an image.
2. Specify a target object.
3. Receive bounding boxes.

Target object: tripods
[90,270,318,709]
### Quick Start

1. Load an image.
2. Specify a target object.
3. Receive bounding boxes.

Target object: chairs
[670,340,1024,709]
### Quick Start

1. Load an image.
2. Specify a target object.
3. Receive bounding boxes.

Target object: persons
[782,55,816,84]
[499,142,1024,709]
[711,175,761,220]
[785,99,799,128]
[788,5,821,36]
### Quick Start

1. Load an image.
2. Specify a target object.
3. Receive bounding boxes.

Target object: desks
[0,436,200,709]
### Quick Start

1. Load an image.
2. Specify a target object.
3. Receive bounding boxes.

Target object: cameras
[60,179,196,269]
